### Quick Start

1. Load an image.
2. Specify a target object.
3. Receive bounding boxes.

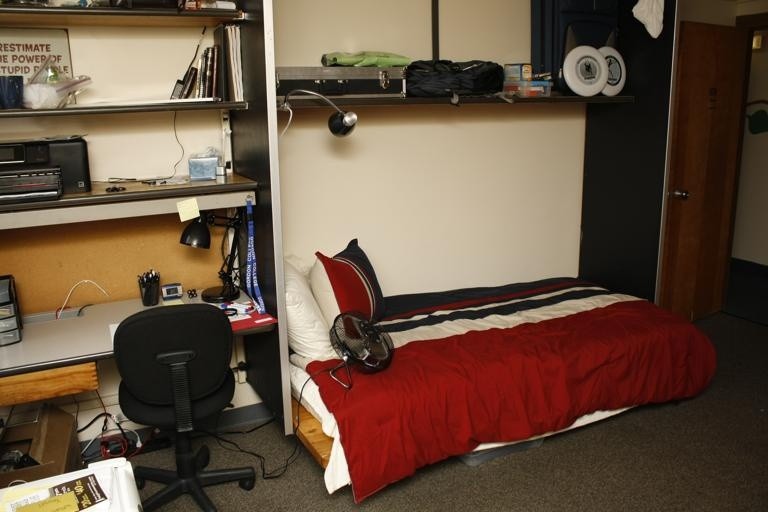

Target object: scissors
[106,185,124,191]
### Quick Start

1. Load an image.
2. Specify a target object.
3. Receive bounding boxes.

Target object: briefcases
[275,66,408,98]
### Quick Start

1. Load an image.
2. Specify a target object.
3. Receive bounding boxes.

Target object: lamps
[282,89,357,137]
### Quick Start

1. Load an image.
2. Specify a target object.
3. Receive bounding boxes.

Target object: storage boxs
[0,401,82,488]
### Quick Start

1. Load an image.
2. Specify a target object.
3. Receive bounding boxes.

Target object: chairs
[113,303,256,512]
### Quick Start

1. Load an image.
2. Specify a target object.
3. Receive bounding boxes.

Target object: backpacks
[406,60,505,95]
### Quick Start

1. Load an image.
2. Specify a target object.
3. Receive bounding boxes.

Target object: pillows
[284,238,380,360]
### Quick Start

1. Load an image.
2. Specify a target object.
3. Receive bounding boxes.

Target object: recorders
[0,134,92,192]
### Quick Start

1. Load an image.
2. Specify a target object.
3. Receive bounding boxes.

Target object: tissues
[189,146,223,180]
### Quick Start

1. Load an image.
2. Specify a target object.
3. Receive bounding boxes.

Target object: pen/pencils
[140,269,160,306]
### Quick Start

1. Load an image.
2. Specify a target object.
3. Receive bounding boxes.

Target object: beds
[291,276,709,491]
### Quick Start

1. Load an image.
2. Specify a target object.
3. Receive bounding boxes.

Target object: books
[179,23,245,102]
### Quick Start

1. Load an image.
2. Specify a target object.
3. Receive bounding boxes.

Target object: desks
[1,287,276,462]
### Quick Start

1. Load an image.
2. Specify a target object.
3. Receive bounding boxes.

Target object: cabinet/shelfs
[2,2,259,229]
